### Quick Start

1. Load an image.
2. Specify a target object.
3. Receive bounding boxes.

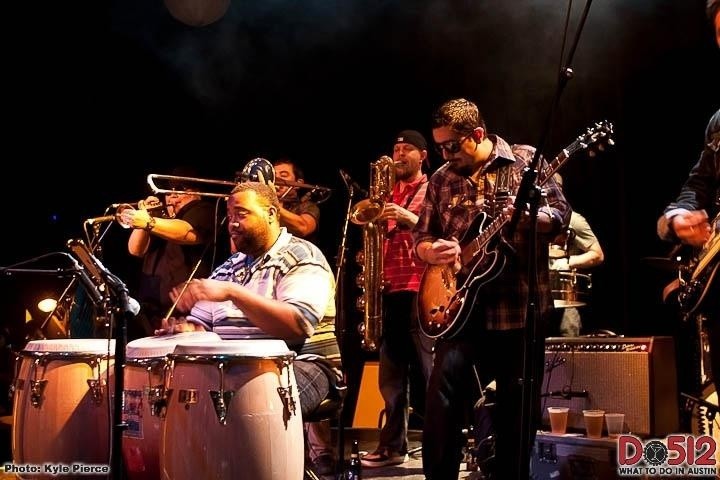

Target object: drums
[157,343,304,480]
[10,339,115,480]
[545,268,593,310]
[123,332,222,479]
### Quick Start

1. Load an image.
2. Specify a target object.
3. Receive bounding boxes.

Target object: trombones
[147,159,332,205]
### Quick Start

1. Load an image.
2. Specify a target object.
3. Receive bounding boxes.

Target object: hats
[394,128,430,151]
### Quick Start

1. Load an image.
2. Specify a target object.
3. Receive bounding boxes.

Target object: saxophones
[350,156,403,351]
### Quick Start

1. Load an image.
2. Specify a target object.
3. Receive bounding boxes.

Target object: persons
[116,98,719,480]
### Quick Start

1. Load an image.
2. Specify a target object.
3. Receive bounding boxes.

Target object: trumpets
[117,202,178,228]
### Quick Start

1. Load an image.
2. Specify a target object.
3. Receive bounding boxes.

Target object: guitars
[417,120,616,341]
[670,209,718,318]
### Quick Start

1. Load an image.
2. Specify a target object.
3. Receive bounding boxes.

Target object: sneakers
[360,450,410,469]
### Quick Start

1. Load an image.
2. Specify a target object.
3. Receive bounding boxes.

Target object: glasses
[163,184,201,196]
[438,125,479,155]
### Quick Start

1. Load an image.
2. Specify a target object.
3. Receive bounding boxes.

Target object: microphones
[71,261,104,308]
[81,243,140,315]
[547,390,588,398]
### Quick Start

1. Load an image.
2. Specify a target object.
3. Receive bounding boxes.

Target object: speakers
[543,335,676,434]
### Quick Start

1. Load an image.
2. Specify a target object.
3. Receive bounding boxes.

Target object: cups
[548,406,569,437]
[605,414,625,439]
[584,408,605,438]
